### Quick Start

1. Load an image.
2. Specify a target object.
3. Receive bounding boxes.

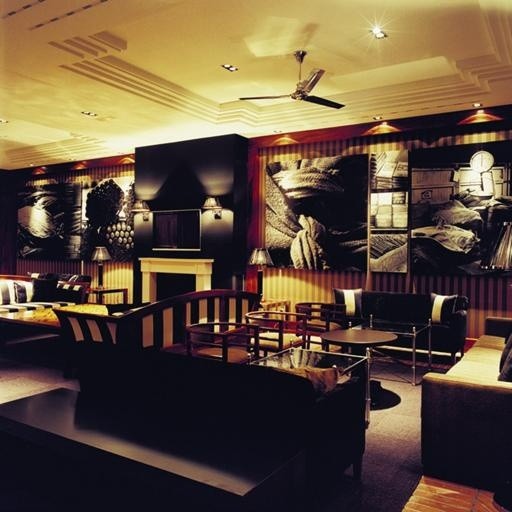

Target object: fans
[237,51,347,109]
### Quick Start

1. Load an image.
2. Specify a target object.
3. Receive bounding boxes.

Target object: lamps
[92,246,113,289]
[131,199,150,222]
[203,198,223,218]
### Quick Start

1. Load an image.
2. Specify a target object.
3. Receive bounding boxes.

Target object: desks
[83,288,129,307]
[320,329,398,431]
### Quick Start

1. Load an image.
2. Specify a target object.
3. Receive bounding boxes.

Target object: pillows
[497,328,511,381]
[27,272,92,300]
[333,288,365,317]
[428,293,456,323]
[1,279,29,306]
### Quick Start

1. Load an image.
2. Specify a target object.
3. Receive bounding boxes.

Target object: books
[376,176,408,190]
[480,222,512,270]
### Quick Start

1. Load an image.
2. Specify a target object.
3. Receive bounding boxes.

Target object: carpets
[1,339,422,512]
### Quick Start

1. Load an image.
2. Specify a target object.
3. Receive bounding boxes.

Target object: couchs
[329,291,469,364]
[420,315,511,494]
[0,274,91,372]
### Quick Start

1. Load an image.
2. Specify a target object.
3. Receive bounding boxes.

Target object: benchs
[1,387,320,510]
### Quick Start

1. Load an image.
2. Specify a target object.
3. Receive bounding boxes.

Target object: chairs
[245,309,309,361]
[297,301,352,374]
[187,322,260,370]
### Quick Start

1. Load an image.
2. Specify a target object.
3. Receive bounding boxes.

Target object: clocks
[469,150,494,173]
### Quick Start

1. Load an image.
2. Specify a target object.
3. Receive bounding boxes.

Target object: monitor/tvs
[152,209,202,252]
